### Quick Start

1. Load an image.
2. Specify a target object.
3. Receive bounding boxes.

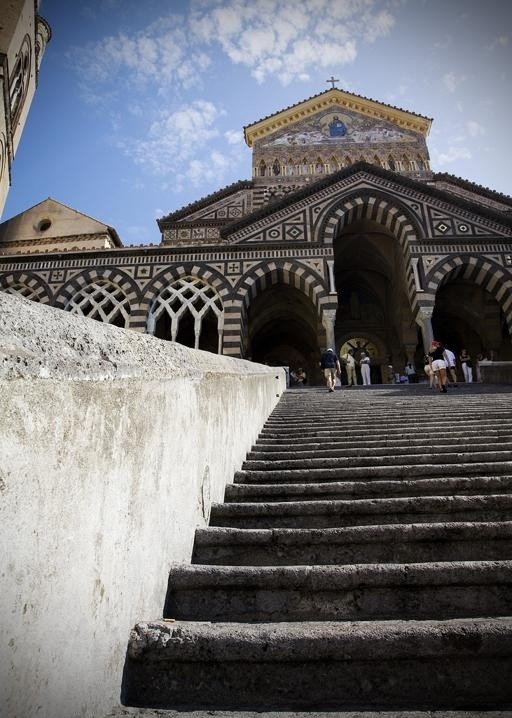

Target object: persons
[294,339,482,393]
[329,115,347,136]
